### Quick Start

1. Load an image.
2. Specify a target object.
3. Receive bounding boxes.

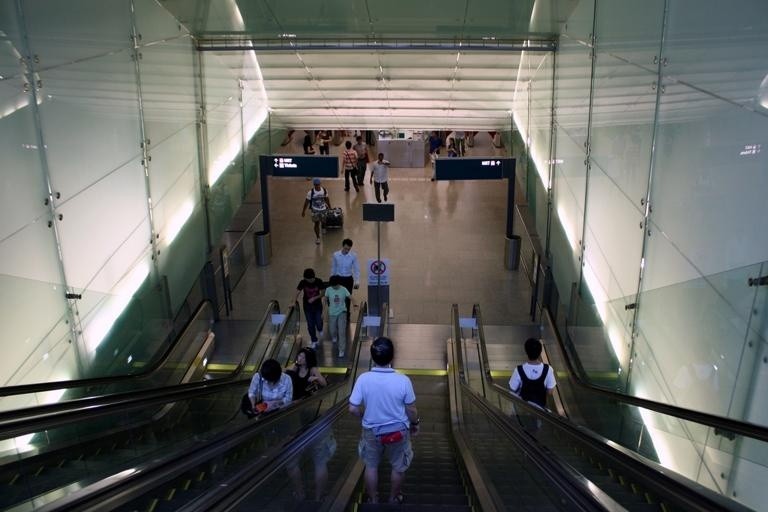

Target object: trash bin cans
[253,230,273,267]
[504,234,522,270]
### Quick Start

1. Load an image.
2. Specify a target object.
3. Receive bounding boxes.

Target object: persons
[287,268,326,349]
[349,335,422,505]
[283,346,328,403]
[312,233,328,275]
[428,179,441,222]
[342,190,363,235]
[507,335,557,439]
[447,178,457,218]
[301,178,332,245]
[282,422,337,506]
[249,358,295,414]
[322,274,358,358]
[330,237,361,327]
[302,129,466,203]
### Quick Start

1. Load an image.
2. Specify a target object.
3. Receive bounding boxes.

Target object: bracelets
[409,418,421,427]
[353,305,358,308]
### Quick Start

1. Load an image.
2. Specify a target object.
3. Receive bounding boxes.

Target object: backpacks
[515,363,551,406]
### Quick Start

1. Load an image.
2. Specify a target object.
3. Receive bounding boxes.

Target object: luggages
[322,207,343,228]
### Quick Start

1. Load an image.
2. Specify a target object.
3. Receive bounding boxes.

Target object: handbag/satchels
[241,393,257,417]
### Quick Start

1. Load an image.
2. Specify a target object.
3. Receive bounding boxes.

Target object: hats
[314,179,320,185]
